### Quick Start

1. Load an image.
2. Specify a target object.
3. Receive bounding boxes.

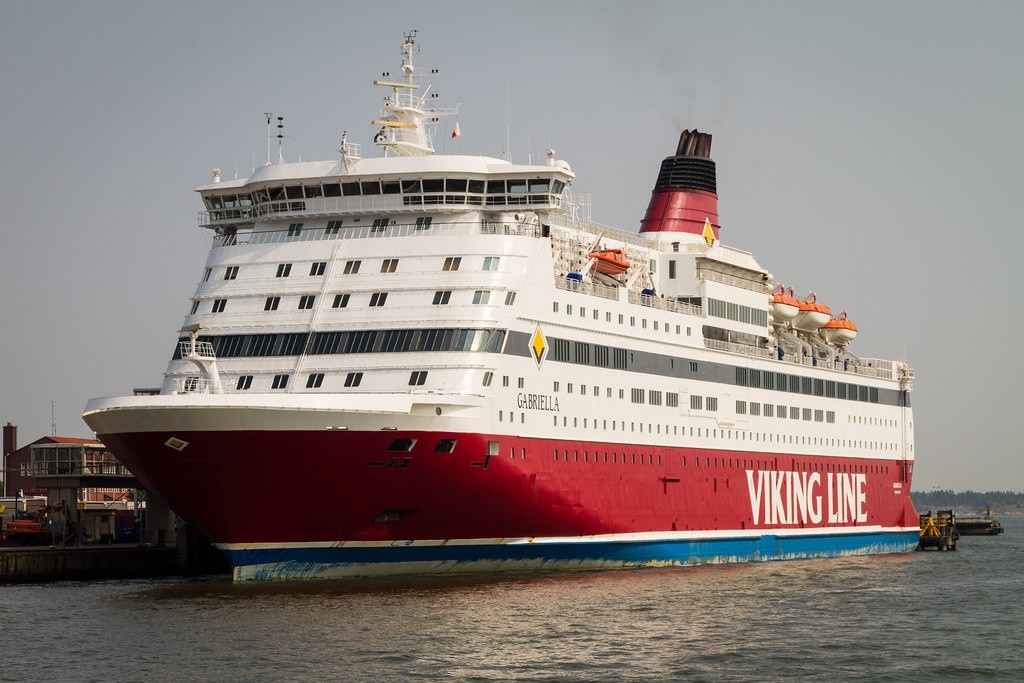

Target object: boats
[919,505,1005,535]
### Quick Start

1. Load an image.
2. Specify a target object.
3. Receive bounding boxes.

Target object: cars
[114,527,138,543]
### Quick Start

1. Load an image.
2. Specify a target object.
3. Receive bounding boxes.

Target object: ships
[82,29,922,583]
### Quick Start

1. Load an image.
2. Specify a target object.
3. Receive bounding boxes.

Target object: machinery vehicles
[7,499,78,546]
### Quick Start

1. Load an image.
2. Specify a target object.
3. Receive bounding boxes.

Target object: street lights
[4,452,9,498]
[15,488,23,519]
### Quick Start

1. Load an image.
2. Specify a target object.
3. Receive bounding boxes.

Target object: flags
[452,122,460,138]
